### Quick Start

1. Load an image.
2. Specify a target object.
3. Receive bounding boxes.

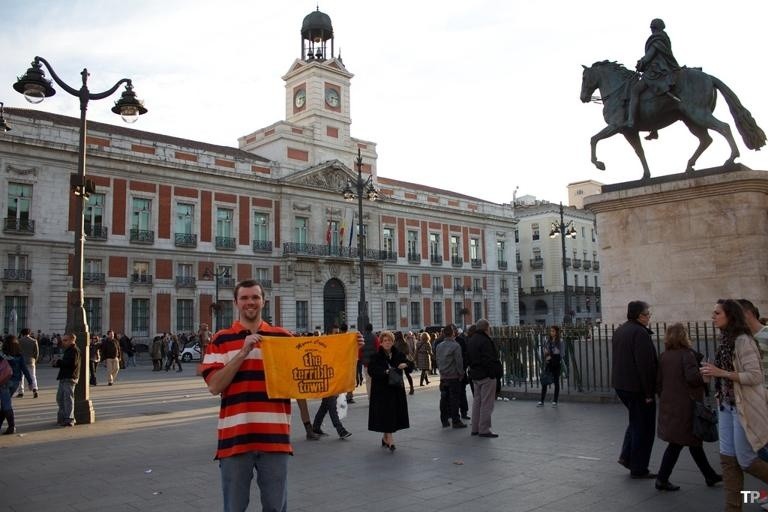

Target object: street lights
[550,201,577,339]
[341,146,378,354]
[10,54,146,426]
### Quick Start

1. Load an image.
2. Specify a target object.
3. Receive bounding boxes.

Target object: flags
[349,220,354,247]
[338,217,346,244]
[326,212,332,246]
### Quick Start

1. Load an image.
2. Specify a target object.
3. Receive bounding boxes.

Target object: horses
[580,60,768,182]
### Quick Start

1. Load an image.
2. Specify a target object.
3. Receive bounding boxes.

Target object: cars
[176,341,206,362]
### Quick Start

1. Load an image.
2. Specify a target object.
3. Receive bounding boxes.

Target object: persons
[621,17,686,142]
[698,299,767,511]
[611,299,659,478]
[288,318,591,451]
[1,327,210,435]
[735,299,767,389]
[199,279,365,511]
[654,321,722,495]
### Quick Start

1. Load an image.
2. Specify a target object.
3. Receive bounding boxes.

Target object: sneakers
[33,392,37,397]
[552,402,557,407]
[471,430,499,438]
[339,431,352,438]
[6,427,16,433]
[461,415,471,419]
[16,393,24,397]
[537,402,544,407]
[452,422,467,428]
[305,429,328,440]
[442,421,451,427]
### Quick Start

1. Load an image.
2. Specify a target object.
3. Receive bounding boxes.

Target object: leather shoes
[631,472,658,479]
[706,475,722,487]
[655,480,680,490]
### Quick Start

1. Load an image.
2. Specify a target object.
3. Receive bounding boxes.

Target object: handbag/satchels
[687,402,718,442]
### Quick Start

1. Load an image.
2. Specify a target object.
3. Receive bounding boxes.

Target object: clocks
[325,87,340,109]
[293,89,306,109]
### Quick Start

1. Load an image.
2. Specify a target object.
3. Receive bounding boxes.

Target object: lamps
[93,191,102,208]
[140,183,149,213]
[260,214,266,226]
[224,209,232,224]
[184,204,191,218]
[18,184,25,200]
